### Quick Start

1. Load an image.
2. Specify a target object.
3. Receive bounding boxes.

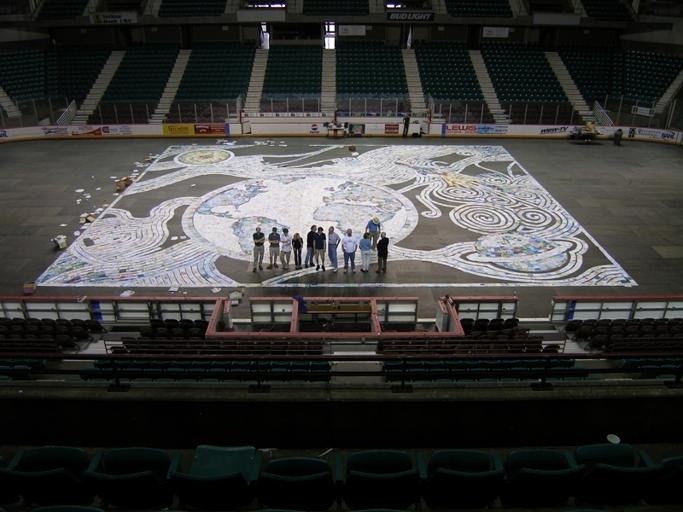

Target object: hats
[372,216,380,225]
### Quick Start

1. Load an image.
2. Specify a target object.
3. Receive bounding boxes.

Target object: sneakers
[274,263,278,268]
[305,262,315,268]
[342,269,348,273]
[295,266,303,270]
[352,268,356,273]
[267,264,273,269]
[361,267,369,272]
[316,264,320,271]
[321,265,326,272]
[332,267,338,273]
[253,268,257,272]
[280,264,289,270]
[376,267,385,274]
[259,265,263,270]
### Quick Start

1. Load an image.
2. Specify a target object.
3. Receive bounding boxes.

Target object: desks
[328,127,343,137]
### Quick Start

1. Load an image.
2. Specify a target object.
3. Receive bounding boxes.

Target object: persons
[365,217,380,250]
[290,232,303,270]
[311,227,327,272]
[266,227,279,270]
[252,226,264,272]
[374,231,389,275]
[583,121,594,142]
[357,232,370,272]
[304,224,316,268]
[401,114,409,137]
[326,226,340,274]
[340,229,357,273]
[277,227,291,270]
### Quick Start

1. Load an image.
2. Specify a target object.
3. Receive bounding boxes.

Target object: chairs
[335,451,416,512]
[310,125,319,136]
[171,445,250,512]
[0,450,16,472]
[85,448,171,512]
[0,447,85,512]
[576,443,654,512]
[501,448,580,512]
[637,449,683,512]
[416,450,501,512]
[250,450,335,512]
